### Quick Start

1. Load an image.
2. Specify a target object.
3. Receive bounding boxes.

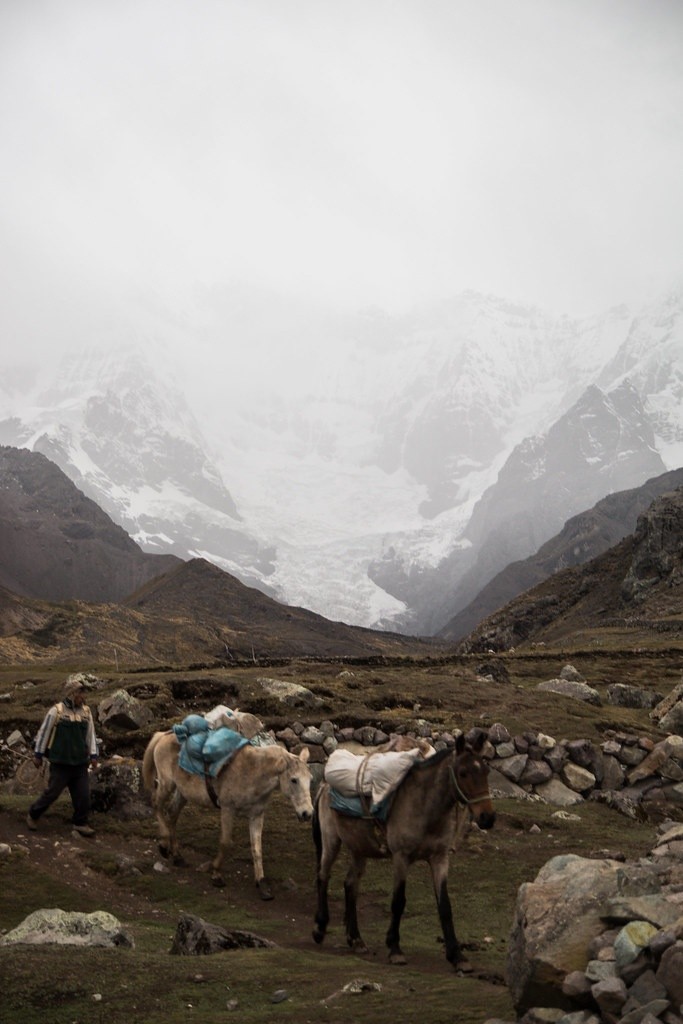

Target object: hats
[64,672,98,697]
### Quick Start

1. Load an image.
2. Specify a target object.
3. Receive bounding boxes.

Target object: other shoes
[72,824,96,835]
[25,813,37,831]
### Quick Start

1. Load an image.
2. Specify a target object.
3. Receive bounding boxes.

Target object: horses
[313,731,497,972]
[142,730,315,900]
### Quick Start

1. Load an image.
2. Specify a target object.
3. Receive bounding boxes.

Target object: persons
[26,680,98,834]
